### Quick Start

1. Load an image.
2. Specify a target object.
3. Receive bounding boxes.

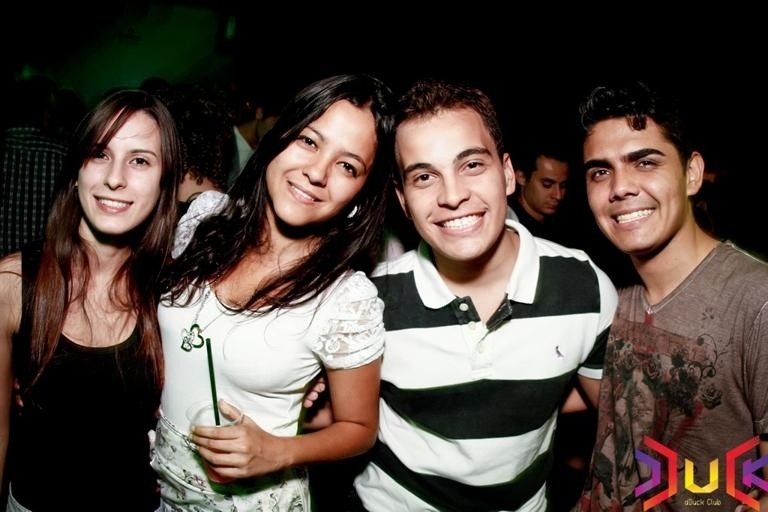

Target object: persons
[127,70,399,512]
[234,88,288,173]
[295,83,620,511]
[511,148,569,238]
[156,87,238,219]
[0,88,189,511]
[580,80,768,511]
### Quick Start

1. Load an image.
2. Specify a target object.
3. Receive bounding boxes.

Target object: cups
[185,399,244,483]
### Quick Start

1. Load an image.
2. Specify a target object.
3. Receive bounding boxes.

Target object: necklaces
[180,277,254,347]
